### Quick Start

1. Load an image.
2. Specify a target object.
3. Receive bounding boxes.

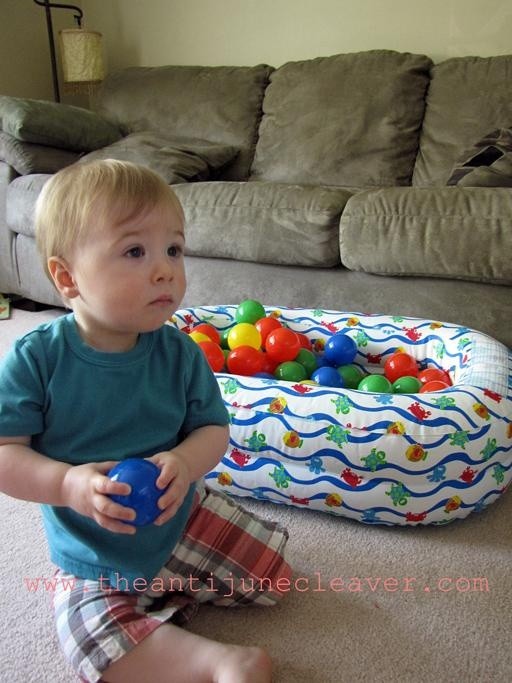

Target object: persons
[1,158,295,682]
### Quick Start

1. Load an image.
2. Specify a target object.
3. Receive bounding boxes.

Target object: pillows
[0,96,242,187]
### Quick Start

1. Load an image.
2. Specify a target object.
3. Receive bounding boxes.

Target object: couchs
[0,48,512,349]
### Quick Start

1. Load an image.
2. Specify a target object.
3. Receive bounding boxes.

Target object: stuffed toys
[183,300,457,395]
[102,457,162,527]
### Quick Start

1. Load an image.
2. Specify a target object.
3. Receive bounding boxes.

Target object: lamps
[32,0,106,102]
